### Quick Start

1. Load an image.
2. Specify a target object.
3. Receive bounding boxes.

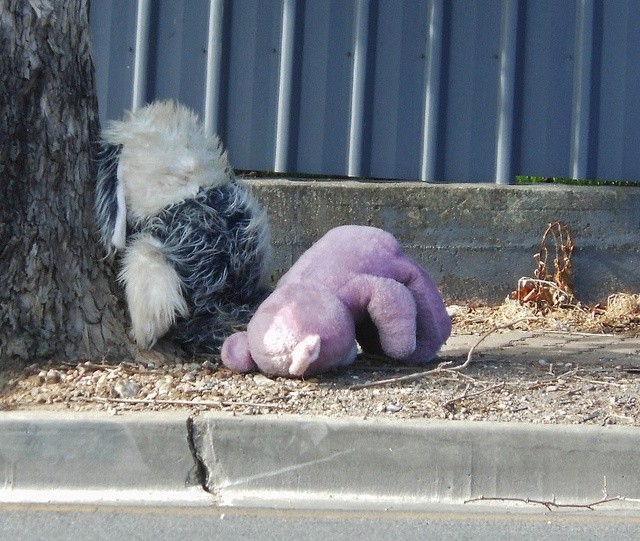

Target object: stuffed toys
[219,224,453,380]
[94,96,274,356]
